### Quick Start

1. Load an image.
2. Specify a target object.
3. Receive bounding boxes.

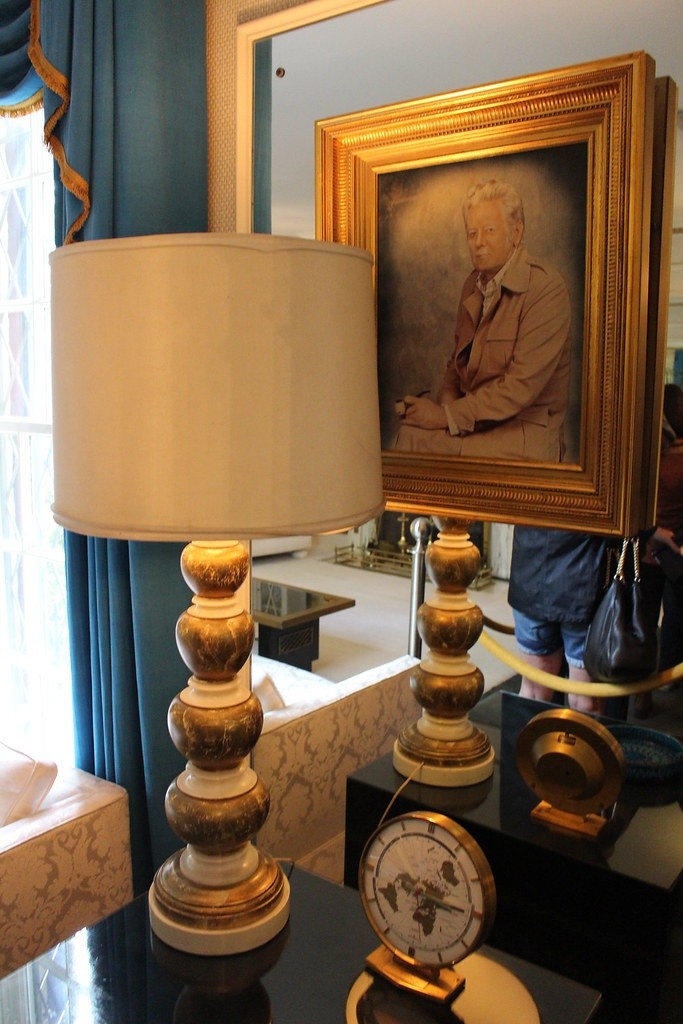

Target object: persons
[508,524,683,722]
[633,384,683,719]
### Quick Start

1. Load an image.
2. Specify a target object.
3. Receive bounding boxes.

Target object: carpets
[312,634,397,684]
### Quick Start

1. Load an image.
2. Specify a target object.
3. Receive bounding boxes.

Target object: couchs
[251,654,423,886]
[0,741,131,983]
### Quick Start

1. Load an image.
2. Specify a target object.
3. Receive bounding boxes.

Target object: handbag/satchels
[585,536,665,684]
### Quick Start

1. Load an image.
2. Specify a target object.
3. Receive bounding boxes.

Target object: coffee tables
[250,573,356,672]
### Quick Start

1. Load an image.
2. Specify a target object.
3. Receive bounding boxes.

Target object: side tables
[0,859,603,1024]
[345,689,683,1024]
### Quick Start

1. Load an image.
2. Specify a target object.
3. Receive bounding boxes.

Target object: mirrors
[206,1,683,1024]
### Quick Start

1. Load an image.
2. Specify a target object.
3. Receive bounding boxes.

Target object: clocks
[515,706,621,840]
[359,810,497,1021]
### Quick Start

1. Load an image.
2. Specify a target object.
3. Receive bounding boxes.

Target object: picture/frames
[311,53,655,538]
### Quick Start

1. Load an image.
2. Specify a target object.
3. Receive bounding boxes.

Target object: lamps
[55,231,387,961]
[393,514,497,789]
